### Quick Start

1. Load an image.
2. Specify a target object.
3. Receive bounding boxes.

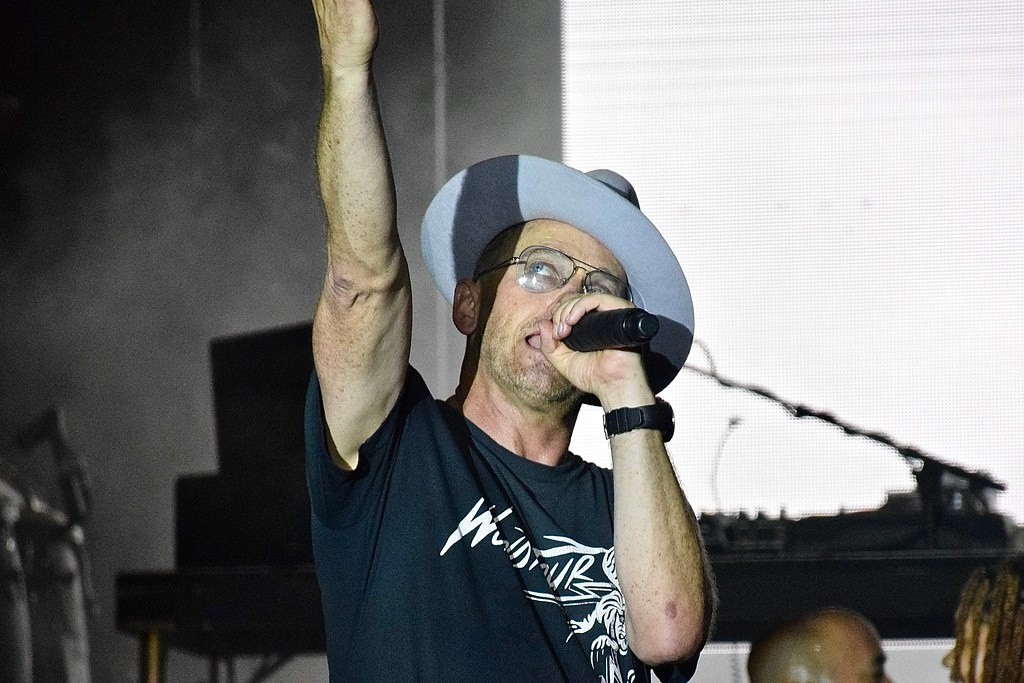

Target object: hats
[421,154,693,404]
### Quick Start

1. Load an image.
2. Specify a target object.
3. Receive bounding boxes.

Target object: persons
[304,0,717,683]
[746,557,1024,683]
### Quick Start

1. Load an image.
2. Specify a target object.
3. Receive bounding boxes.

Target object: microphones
[562,307,660,353]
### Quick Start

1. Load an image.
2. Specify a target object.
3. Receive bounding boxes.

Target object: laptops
[206,320,316,470]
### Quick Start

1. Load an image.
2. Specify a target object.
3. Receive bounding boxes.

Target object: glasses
[475,245,632,309]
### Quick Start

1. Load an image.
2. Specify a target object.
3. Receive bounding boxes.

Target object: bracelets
[602,396,675,444]
